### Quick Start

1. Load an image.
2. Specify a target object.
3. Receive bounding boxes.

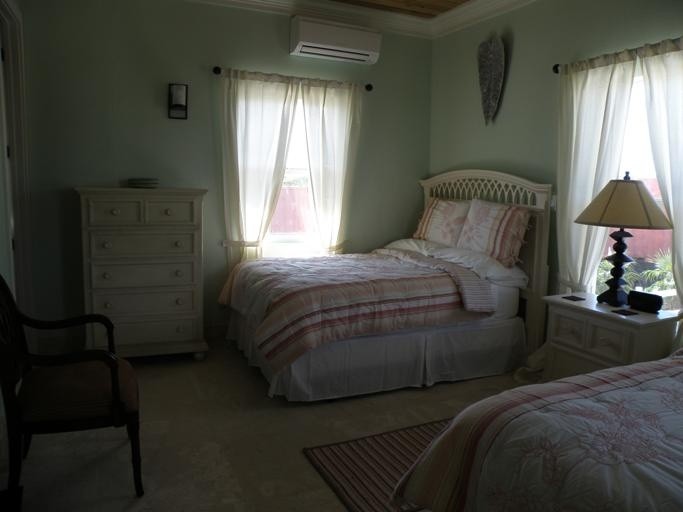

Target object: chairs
[0,274,145,499]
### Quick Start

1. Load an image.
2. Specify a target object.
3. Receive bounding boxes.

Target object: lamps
[574,172,673,307]
[168,82,188,120]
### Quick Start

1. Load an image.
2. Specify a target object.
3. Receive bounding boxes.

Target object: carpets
[302,416,458,512]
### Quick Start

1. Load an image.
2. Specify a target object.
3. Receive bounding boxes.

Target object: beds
[391,347,683,512]
[219,169,553,402]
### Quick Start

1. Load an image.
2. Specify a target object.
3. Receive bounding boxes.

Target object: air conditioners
[289,15,382,65]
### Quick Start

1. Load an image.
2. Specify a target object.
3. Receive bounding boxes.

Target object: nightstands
[540,292,680,375]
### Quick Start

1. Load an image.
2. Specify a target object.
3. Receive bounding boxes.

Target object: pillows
[457,199,530,268]
[414,197,470,249]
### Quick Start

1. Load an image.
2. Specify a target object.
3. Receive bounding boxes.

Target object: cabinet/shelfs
[74,186,210,360]
